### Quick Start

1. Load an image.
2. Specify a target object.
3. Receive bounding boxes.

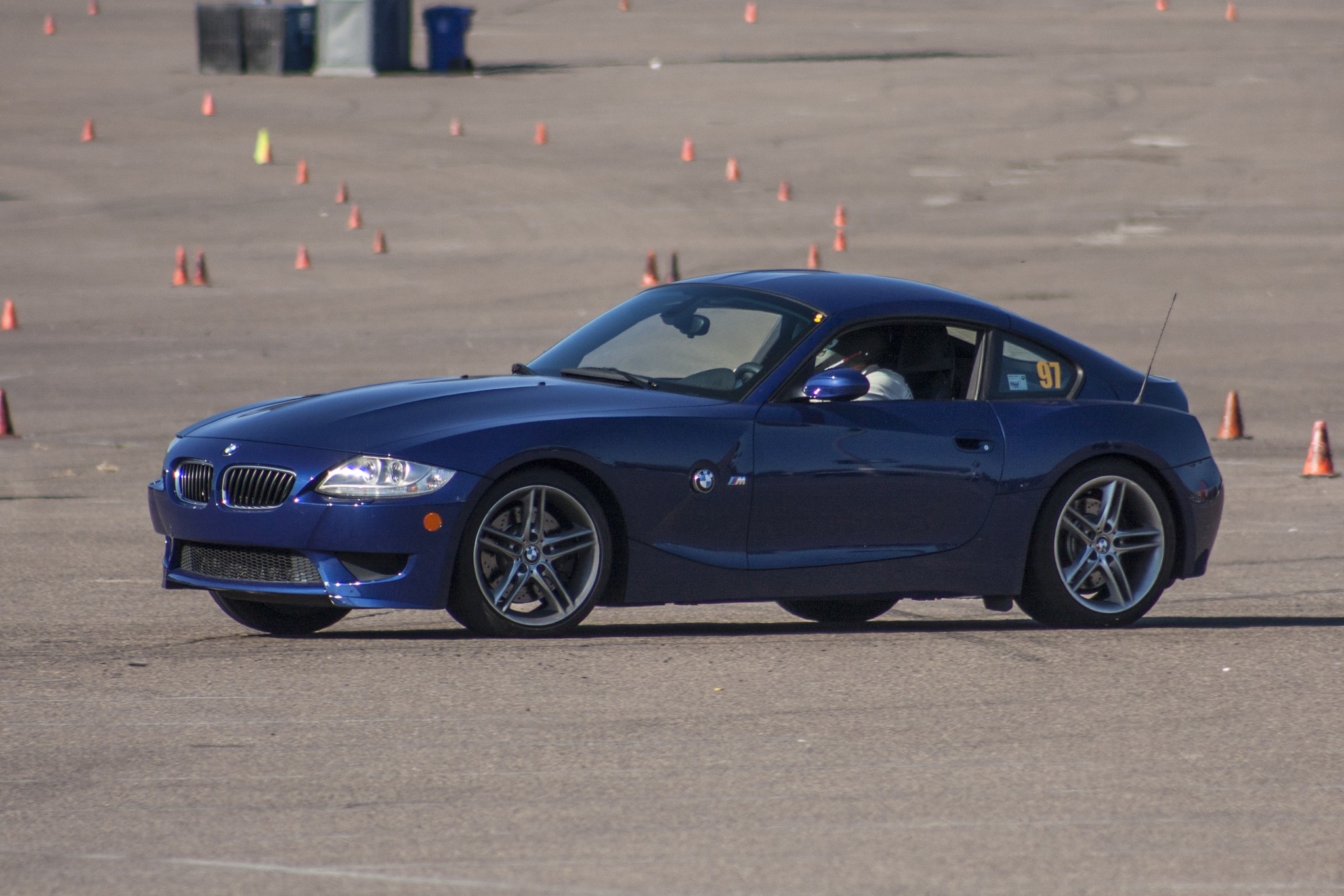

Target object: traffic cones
[531,122,551,143]
[1211,389,1253,441]
[332,183,348,201]
[295,161,308,185]
[345,204,360,230]
[42,8,56,35]
[250,126,271,166]
[1299,420,1339,478]
[742,0,762,23]
[89,2,99,16]
[0,386,20,441]
[295,248,312,271]
[805,249,821,271]
[641,248,680,293]
[1154,0,1170,11]
[833,208,849,252]
[1224,3,1241,24]
[0,299,18,329]
[81,117,94,141]
[679,138,696,161]
[778,182,790,202]
[449,114,467,139]
[370,231,390,255]
[201,93,212,113]
[726,157,738,181]
[171,248,207,286]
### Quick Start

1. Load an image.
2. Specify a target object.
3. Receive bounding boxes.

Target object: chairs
[890,325,957,400]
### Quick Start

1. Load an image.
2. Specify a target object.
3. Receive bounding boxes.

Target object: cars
[147,270,1221,640]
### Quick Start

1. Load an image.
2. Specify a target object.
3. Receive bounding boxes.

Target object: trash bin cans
[422,7,475,70]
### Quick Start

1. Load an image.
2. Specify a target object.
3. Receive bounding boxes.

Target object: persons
[815,325,914,401]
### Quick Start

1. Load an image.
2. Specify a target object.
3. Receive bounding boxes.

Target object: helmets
[829,325,894,363]
